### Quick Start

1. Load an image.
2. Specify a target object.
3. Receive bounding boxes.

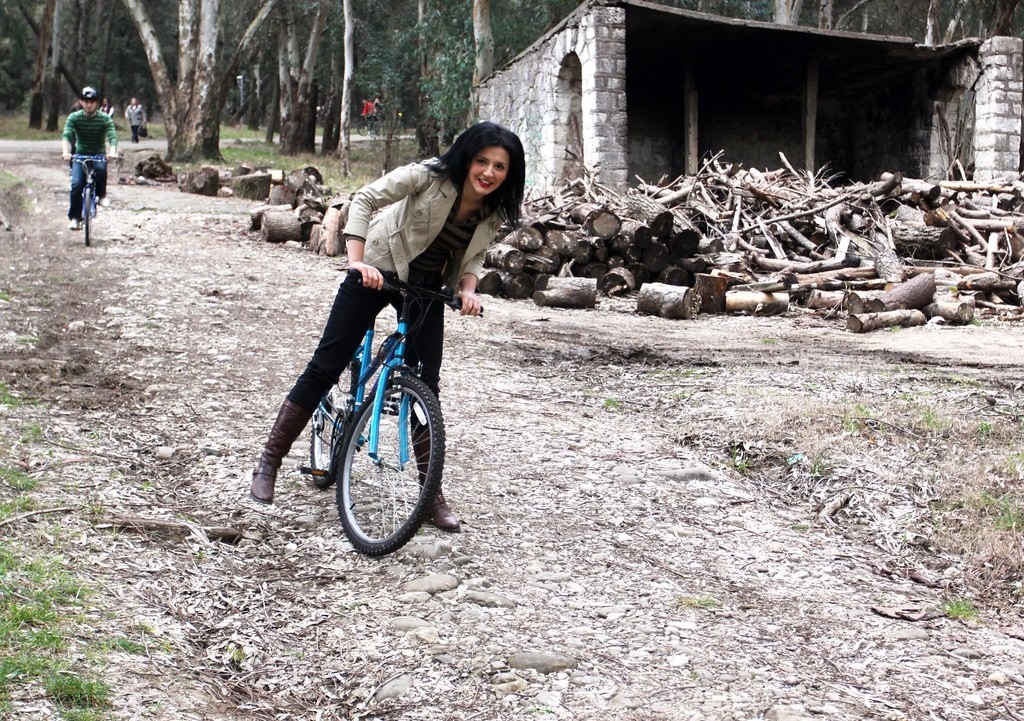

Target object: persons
[100,96,115,119]
[361,96,384,138]
[69,100,82,169]
[251,122,526,531]
[62,87,117,229]
[126,98,147,143]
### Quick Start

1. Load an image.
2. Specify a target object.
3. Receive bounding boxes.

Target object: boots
[250,396,313,505]
[401,427,462,534]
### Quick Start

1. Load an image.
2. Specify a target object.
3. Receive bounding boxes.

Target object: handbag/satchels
[138,126,148,138]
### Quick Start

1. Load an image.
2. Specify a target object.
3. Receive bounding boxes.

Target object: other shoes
[93,198,102,215]
[70,218,79,231]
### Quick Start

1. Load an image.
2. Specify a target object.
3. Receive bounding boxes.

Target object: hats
[81,86,97,102]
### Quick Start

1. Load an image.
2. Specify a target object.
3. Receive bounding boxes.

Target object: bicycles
[62,155,117,248]
[299,268,483,556]
[358,116,386,137]
